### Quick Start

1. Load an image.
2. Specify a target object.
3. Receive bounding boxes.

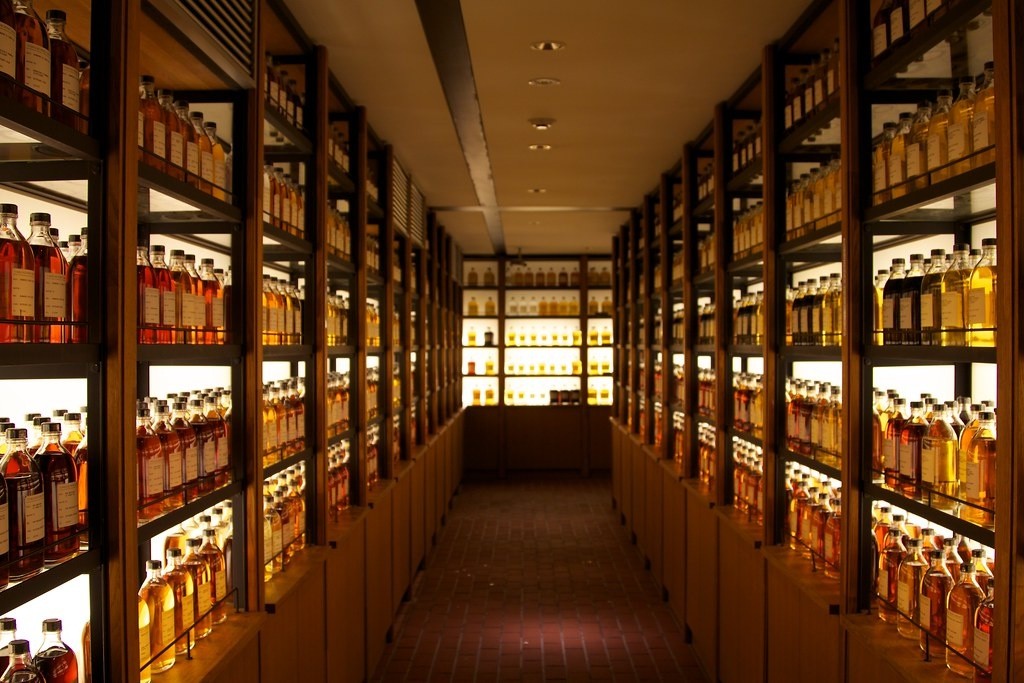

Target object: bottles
[602,326,611,344]
[509,297,517,315]
[697,424,716,493]
[784,39,840,141]
[558,268,569,286]
[538,297,547,314]
[698,303,716,344]
[698,162,714,200]
[589,327,598,345]
[528,296,538,315]
[549,297,558,314]
[733,372,763,438]
[730,291,763,345]
[137,76,232,205]
[732,440,764,526]
[366,366,379,487]
[558,296,568,314]
[466,323,582,405]
[325,371,351,521]
[328,203,351,261]
[0,407,89,589]
[469,297,478,315]
[0,618,91,683]
[786,272,840,346]
[136,387,232,683]
[262,275,302,344]
[589,266,609,283]
[263,376,306,583]
[787,155,840,240]
[264,160,306,239]
[731,118,761,183]
[602,297,611,312]
[733,202,763,260]
[871,386,997,683]
[0,0,89,134]
[784,378,845,582]
[568,297,578,314]
[519,297,527,314]
[468,268,477,284]
[570,268,580,285]
[484,268,495,284]
[485,297,495,315]
[589,297,598,314]
[698,368,716,419]
[506,267,545,286]
[872,0,995,205]
[367,303,379,347]
[366,234,380,274]
[326,294,349,346]
[873,238,998,345]
[138,244,225,343]
[547,268,557,285]
[328,122,352,174]
[265,53,303,144]
[0,202,89,344]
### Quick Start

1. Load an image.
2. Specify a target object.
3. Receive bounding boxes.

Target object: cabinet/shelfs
[462,252,614,481]
[0,0,465,683]
[608,0,1024,683]
